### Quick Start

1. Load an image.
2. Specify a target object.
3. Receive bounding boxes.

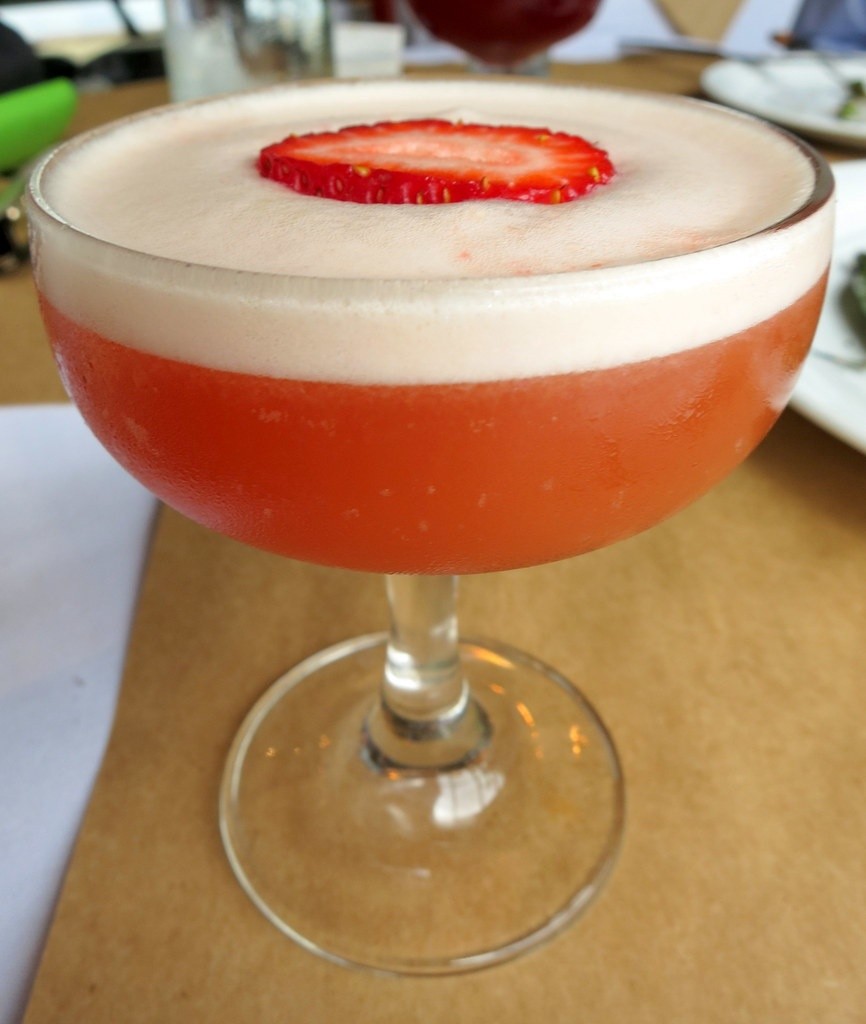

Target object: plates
[698,43,866,152]
[766,162,866,454]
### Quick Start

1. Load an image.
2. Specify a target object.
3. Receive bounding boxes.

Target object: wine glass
[407,0,603,79]
[27,76,835,977]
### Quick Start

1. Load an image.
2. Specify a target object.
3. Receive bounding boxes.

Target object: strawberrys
[254,113,617,205]
